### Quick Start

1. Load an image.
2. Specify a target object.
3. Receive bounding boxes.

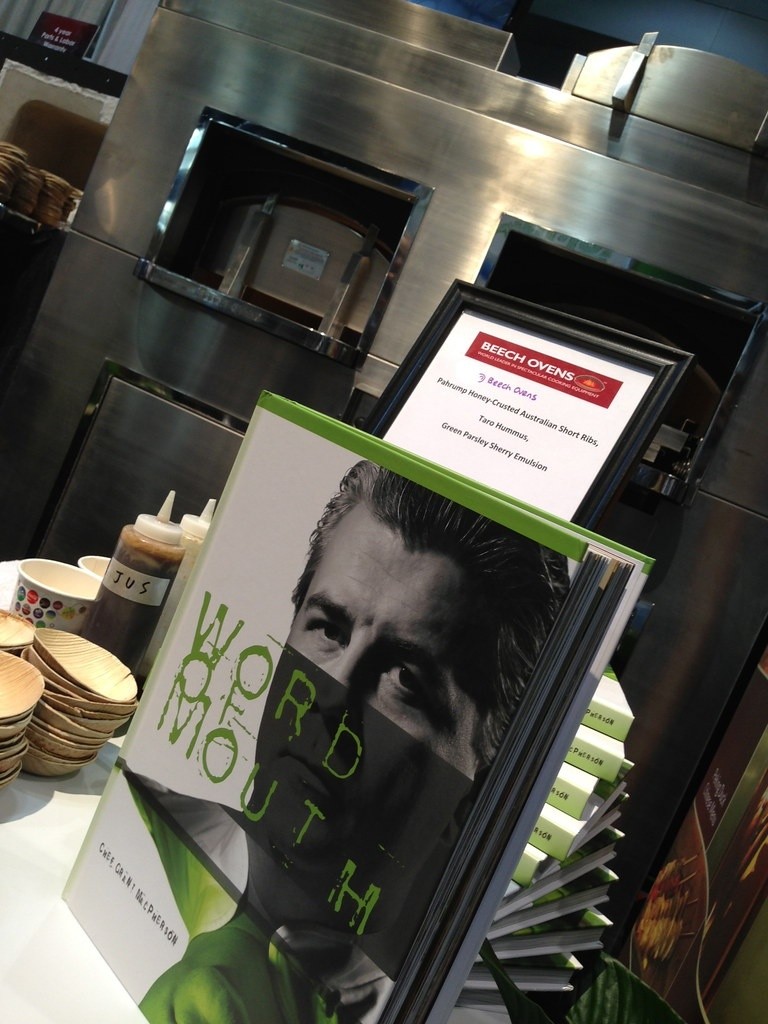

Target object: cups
[11,558,102,635]
[78,556,111,580]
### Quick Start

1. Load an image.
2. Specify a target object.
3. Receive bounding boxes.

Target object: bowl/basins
[0,609,138,791]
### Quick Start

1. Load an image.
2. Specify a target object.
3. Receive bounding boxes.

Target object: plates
[0,137,84,226]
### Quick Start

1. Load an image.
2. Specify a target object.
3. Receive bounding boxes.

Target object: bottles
[112,499,217,738]
[81,491,186,678]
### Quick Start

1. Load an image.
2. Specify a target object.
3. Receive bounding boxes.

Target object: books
[60,387,656,1024]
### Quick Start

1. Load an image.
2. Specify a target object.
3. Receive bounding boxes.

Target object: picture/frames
[361,277,698,534]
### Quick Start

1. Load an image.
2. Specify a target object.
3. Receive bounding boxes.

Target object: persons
[129,461,572,1024]
